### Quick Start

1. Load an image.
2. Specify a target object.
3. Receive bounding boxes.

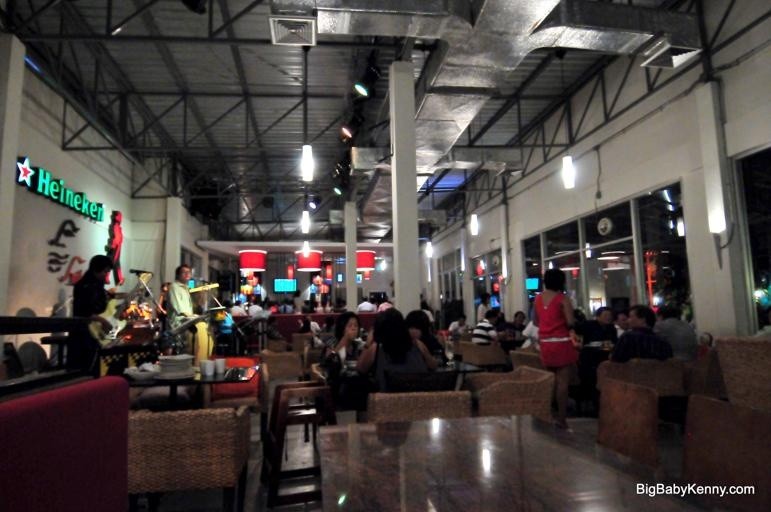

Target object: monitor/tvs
[273,278,299,295]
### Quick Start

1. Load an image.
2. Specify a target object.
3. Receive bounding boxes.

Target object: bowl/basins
[128,371,155,380]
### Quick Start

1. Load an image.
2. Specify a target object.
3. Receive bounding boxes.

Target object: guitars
[88,273,153,349]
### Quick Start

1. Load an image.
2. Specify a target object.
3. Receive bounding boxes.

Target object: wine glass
[445,340,457,366]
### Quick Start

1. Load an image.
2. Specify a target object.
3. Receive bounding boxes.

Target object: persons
[66,245,704,437]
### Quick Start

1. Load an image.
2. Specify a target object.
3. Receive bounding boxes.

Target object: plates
[153,354,197,380]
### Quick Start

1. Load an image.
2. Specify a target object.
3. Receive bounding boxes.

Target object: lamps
[300,43,581,260]
[712,220,738,271]
[238,249,270,272]
[296,250,326,272]
[358,250,377,272]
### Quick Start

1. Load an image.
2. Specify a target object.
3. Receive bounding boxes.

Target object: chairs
[0,332,771,512]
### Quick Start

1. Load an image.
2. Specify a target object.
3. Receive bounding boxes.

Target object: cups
[199,358,226,376]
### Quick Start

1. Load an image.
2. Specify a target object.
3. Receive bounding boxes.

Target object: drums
[116,302,153,321]
[208,305,227,319]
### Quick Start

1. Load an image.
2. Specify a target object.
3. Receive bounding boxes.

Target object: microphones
[199,278,210,283]
[130,269,149,273]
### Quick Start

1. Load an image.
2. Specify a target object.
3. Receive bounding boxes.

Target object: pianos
[181,315,207,324]
